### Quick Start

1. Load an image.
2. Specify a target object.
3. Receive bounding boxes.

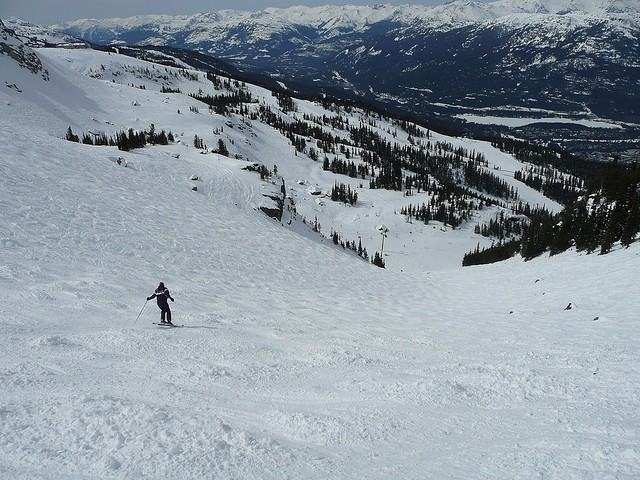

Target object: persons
[147,282,174,324]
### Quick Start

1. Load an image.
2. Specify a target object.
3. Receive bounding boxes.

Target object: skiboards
[153,323,184,327]
